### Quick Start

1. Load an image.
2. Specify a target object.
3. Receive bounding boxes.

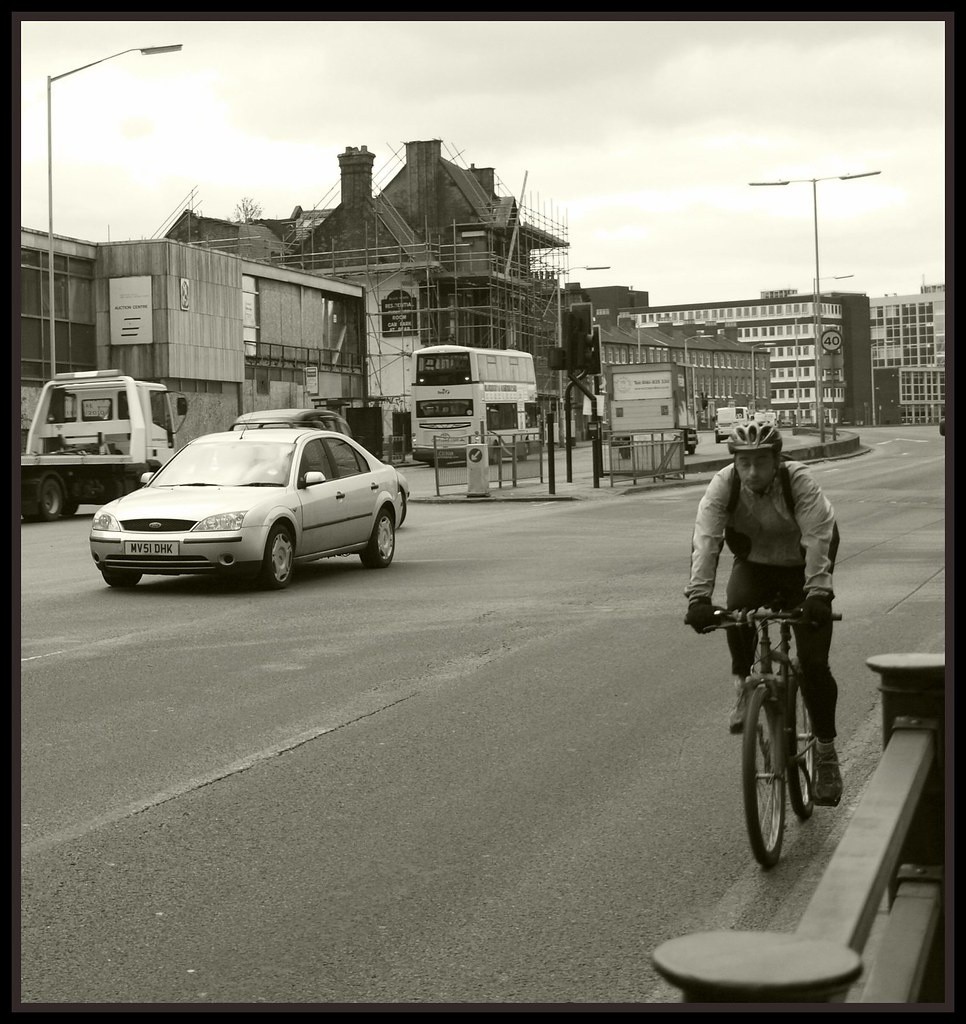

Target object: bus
[409,344,542,466]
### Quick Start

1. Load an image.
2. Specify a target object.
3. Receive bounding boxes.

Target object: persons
[684,422,843,804]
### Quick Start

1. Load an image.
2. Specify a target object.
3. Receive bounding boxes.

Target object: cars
[87,426,411,592]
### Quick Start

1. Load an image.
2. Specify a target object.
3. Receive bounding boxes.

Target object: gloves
[792,596,833,629]
[688,601,714,635]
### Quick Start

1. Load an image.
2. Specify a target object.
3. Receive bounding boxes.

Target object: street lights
[555,264,612,446]
[45,43,184,379]
[811,275,854,423]
[683,335,716,363]
[751,342,777,400]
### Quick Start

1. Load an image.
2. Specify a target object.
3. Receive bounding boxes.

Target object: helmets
[727,422,783,456]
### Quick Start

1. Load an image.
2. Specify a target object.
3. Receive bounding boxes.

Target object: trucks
[609,361,699,453]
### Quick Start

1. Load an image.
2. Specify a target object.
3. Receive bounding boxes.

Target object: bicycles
[684,609,814,868]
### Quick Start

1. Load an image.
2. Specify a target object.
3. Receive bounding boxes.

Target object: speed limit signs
[820,328,844,354]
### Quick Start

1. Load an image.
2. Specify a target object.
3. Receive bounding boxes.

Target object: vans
[713,407,750,444]
[755,412,777,430]
[228,408,354,439]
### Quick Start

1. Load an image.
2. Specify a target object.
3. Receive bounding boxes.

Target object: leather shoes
[812,749,842,807]
[729,678,751,733]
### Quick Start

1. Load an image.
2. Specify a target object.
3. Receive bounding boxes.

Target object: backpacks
[724,464,840,580]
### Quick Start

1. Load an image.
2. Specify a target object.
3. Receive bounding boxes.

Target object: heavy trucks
[22,368,188,522]
